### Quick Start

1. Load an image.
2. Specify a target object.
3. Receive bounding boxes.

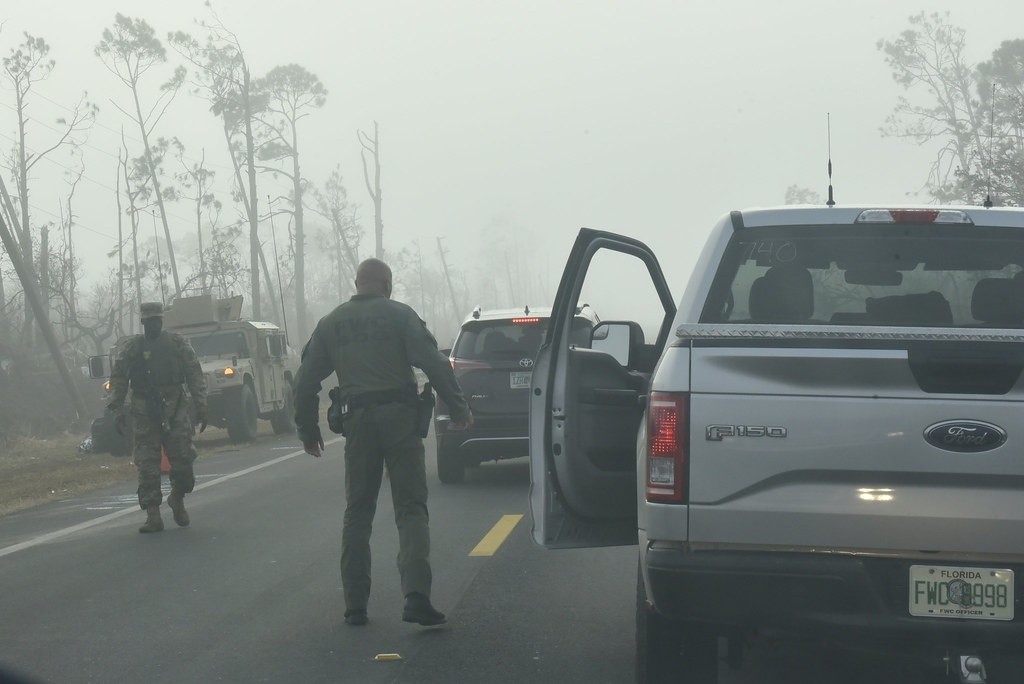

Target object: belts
[342,383,418,410]
[132,390,173,401]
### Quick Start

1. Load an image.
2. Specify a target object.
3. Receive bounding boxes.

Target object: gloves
[446,411,473,431]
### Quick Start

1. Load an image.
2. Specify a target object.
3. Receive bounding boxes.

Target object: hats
[140,301,164,318]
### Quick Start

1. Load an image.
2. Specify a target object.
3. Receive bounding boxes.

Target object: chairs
[475,332,543,364]
[734,261,1024,328]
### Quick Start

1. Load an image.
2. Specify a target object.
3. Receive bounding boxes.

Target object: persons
[108,301,208,533]
[291,258,474,625]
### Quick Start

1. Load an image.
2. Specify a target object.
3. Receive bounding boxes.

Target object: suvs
[432,303,602,484]
[527,198,1024,684]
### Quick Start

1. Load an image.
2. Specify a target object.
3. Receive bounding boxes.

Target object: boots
[167,487,189,526]
[138,504,164,531]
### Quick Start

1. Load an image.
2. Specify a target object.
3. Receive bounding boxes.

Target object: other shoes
[344,607,368,624]
[400,594,448,624]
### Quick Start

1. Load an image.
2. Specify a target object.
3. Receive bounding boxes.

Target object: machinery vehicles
[88,292,297,445]
